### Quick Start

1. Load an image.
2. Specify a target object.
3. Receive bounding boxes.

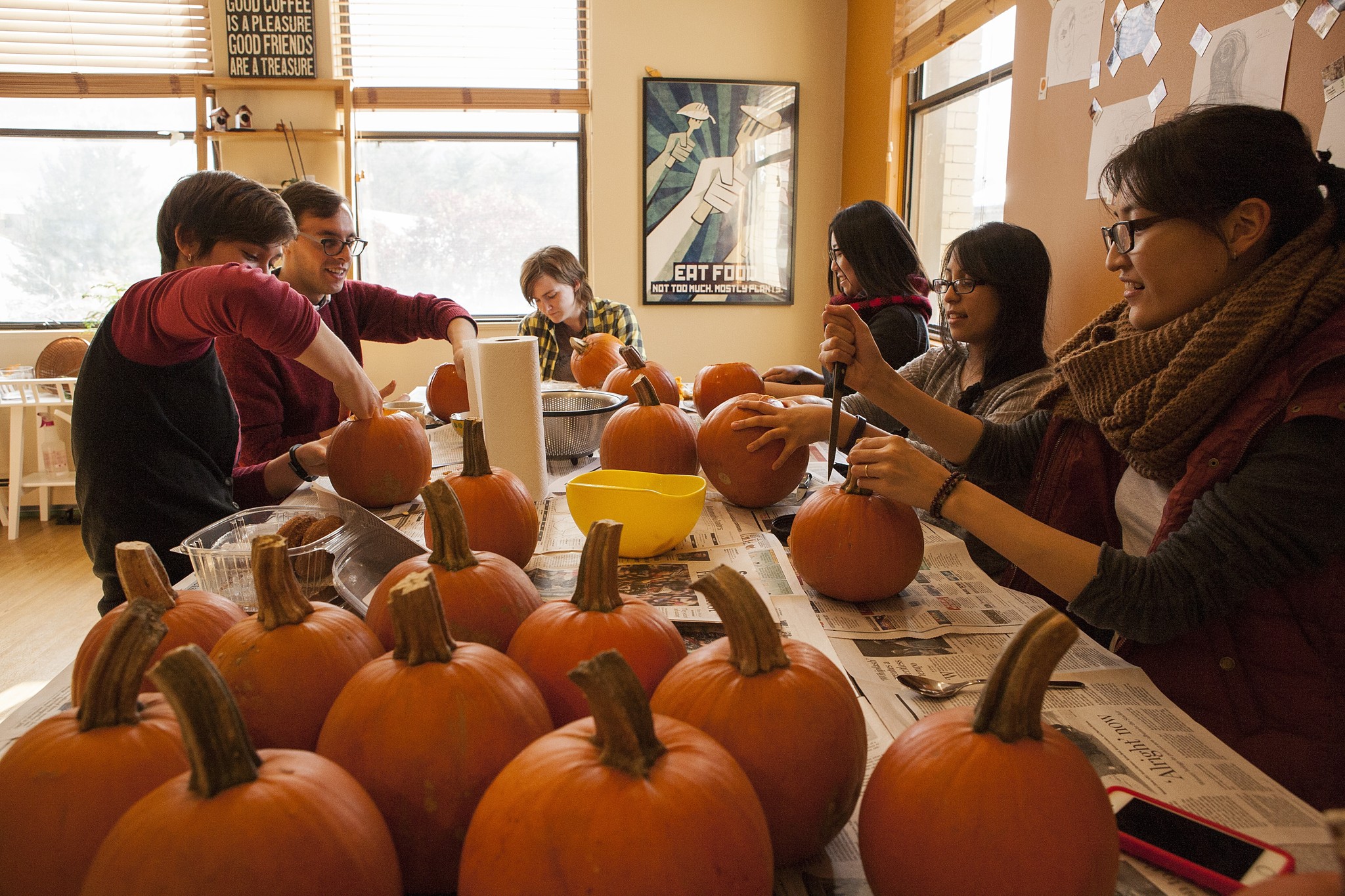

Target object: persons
[516,246,646,382]
[816,104,1345,814]
[730,222,1054,539]
[215,181,477,468]
[69,171,383,618]
[763,201,932,475]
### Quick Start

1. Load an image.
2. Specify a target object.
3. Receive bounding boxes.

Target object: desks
[0,362,89,543]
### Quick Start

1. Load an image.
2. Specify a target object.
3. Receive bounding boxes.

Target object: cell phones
[1106,785,1296,896]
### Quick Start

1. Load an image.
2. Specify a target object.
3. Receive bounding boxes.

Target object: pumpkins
[602,348,681,413]
[364,480,548,656]
[689,391,813,507]
[179,530,383,753]
[458,645,775,896]
[496,517,685,725]
[420,419,540,574]
[848,608,1123,896]
[564,326,625,390]
[689,361,766,418]
[326,404,431,507]
[594,377,702,480]
[788,444,928,608]
[76,639,408,895]
[315,570,553,868]
[1,595,223,896]
[70,536,246,708]
[641,562,867,860]
[423,358,469,421]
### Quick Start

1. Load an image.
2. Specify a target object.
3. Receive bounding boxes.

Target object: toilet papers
[456,334,551,505]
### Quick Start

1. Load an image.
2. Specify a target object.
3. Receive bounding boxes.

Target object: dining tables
[23,376,1343,893]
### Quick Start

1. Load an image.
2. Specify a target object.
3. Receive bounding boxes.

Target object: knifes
[827,362,846,480]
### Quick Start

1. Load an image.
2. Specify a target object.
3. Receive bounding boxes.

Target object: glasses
[932,278,991,294]
[828,248,840,260]
[296,230,368,256]
[1100,214,1183,254]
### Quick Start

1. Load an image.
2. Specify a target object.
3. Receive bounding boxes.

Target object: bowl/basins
[449,410,471,438]
[565,468,707,557]
[382,401,426,420]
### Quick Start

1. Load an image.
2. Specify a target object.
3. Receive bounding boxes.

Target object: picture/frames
[637,75,800,307]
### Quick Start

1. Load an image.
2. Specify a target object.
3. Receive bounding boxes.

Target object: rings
[865,464,871,479]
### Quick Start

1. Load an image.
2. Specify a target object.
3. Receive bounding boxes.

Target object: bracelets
[288,444,320,483]
[929,471,968,520]
[837,414,867,456]
